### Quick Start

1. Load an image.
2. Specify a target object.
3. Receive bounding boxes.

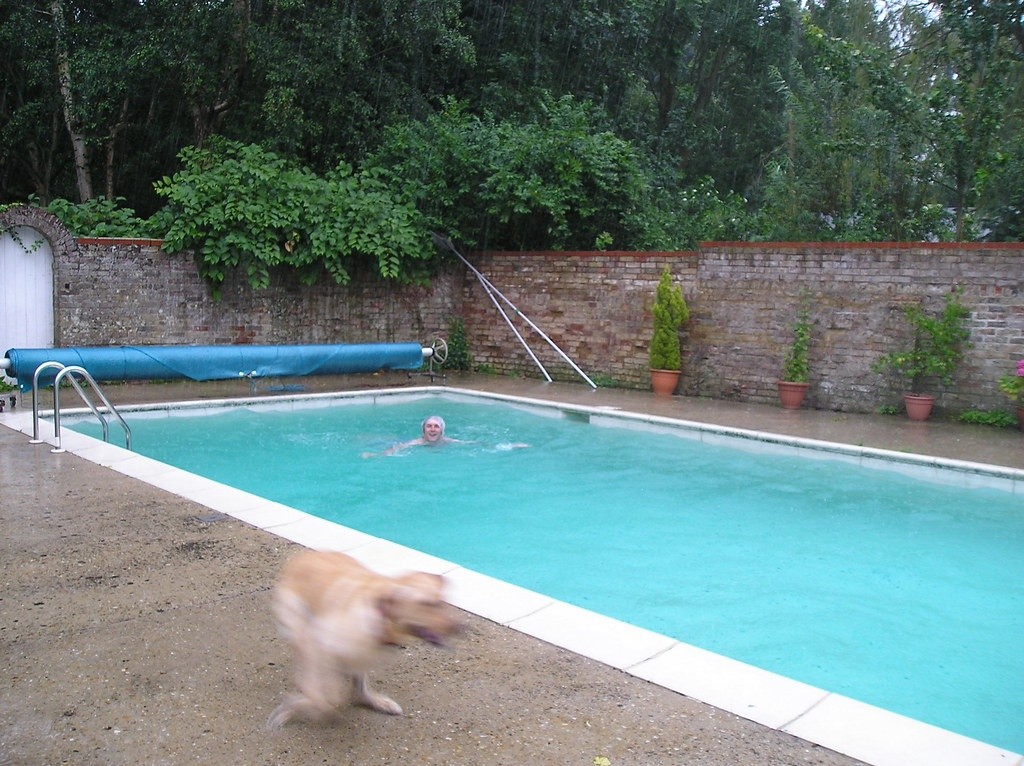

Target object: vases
[1016,408,1024,433]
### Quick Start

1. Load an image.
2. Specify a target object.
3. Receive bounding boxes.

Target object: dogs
[264,549,460,730]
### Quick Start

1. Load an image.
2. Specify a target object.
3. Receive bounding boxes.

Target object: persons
[407,416,458,445]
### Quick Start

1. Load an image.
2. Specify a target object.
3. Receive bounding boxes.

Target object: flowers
[998,358,1024,410]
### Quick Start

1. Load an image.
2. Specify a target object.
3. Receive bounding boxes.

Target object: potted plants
[647,265,690,395]
[871,283,975,421]
[778,289,812,411]
